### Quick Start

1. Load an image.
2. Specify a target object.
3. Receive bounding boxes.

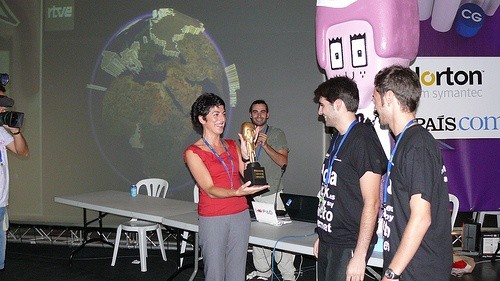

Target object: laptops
[279,193,319,223]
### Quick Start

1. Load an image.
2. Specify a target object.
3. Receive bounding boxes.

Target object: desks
[54,189,384,281]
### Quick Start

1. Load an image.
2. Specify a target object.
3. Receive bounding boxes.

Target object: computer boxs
[461,222,480,252]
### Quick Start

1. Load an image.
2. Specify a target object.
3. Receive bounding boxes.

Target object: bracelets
[12,131,20,135]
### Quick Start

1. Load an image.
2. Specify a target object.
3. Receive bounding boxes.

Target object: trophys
[241,122,268,187]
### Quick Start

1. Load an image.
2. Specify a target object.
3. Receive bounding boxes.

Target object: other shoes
[257,277,268,281]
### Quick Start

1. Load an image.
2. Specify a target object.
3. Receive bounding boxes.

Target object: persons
[184,93,271,281]
[246,100,296,281]
[372,65,453,281]
[314,76,389,281]
[0,85,30,270]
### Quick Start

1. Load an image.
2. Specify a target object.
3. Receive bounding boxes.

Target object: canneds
[130,184,137,197]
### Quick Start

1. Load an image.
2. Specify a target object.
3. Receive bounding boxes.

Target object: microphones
[275,164,288,217]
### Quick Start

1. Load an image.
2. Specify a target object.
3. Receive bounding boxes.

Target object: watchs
[383,268,400,279]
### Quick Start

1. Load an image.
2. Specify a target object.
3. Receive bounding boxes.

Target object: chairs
[449,194,460,233]
[179,183,200,267]
[110,178,169,272]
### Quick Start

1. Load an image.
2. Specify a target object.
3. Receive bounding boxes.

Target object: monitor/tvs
[481,232,500,258]
